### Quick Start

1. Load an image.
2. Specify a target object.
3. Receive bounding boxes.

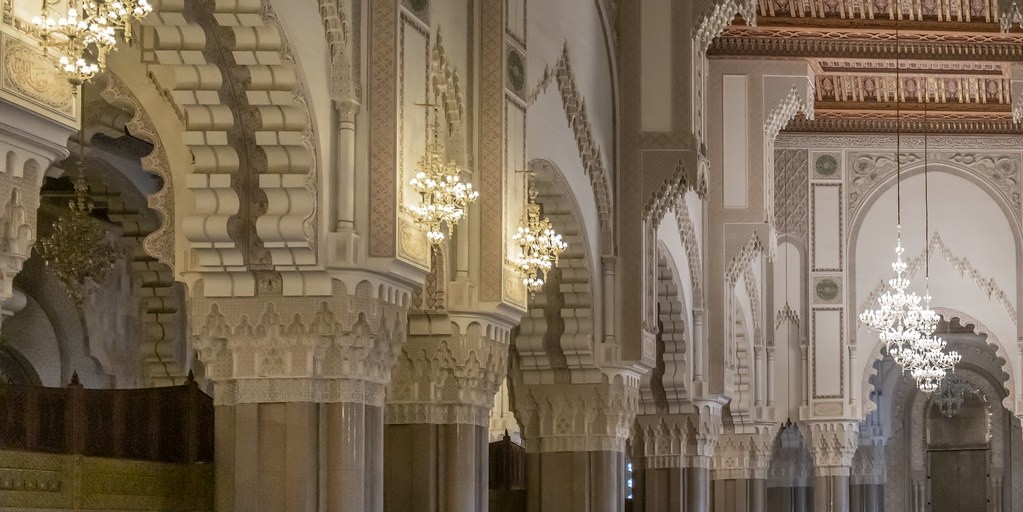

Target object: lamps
[890,0,966,396]
[510,168,568,300]
[856,1,936,355]
[30,0,152,99]
[34,0,122,309]
[926,321,973,417]
[409,102,480,256]
